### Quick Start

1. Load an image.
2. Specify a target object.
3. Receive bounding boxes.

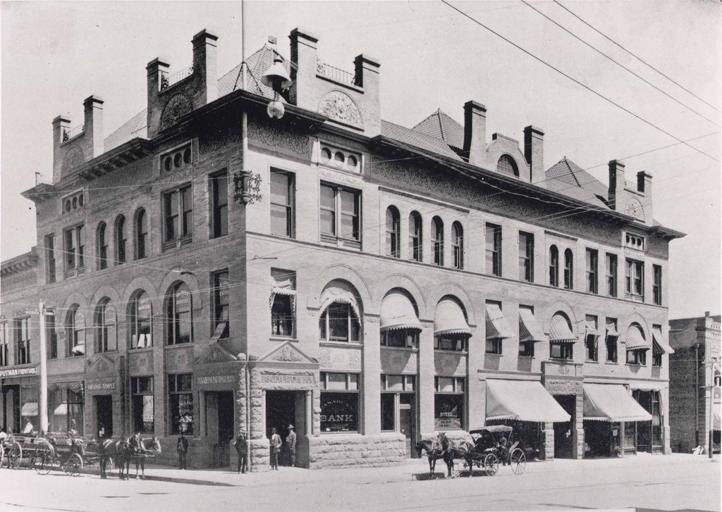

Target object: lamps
[233,170,263,210]
[261,48,299,120]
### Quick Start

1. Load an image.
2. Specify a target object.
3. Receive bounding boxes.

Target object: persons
[271,426,283,470]
[236,434,247,472]
[285,424,296,467]
[177,431,189,469]
[24,418,33,435]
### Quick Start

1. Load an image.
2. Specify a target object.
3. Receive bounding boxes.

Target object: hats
[286,424,294,429]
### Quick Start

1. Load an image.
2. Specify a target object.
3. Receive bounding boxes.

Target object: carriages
[414,425,527,479]
[0,431,162,481]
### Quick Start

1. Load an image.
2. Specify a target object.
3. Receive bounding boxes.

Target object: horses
[436,431,473,479]
[416,439,455,479]
[127,437,163,480]
[99,432,142,479]
